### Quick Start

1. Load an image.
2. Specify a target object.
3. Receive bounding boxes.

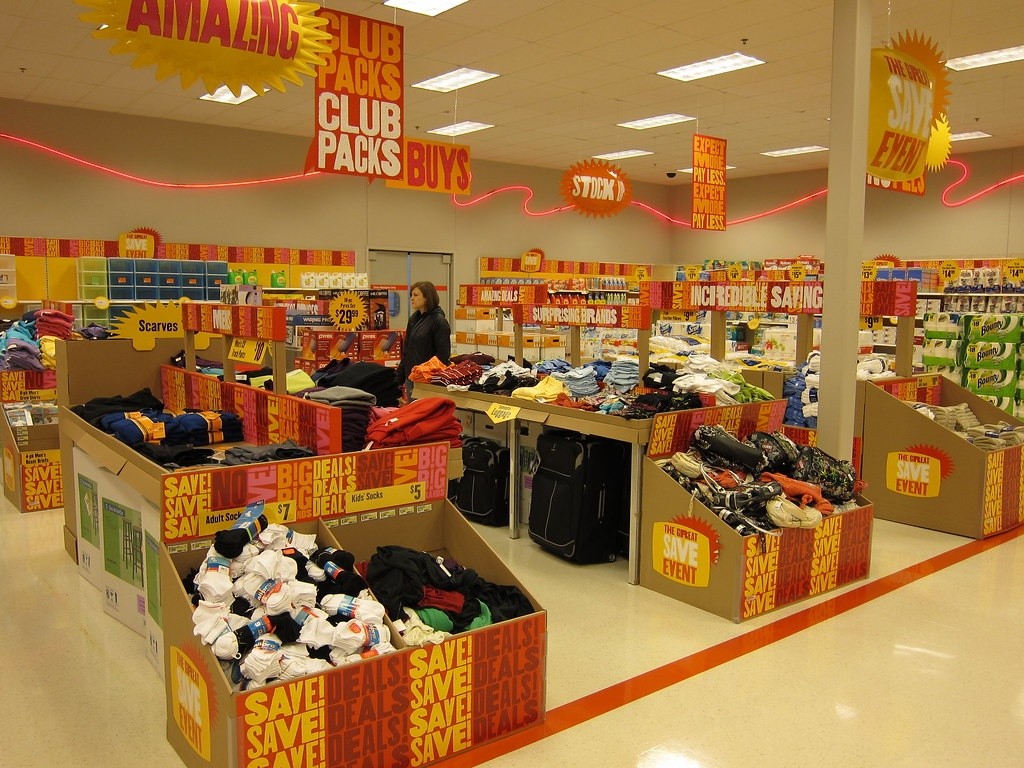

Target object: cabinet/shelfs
[873,294,1024,347]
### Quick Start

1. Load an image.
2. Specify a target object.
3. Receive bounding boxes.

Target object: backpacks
[528,431,616,565]
[458,441,509,526]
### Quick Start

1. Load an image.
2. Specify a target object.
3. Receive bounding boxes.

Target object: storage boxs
[0,259,1024,768]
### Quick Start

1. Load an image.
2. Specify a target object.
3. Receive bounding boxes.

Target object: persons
[396,281,451,376]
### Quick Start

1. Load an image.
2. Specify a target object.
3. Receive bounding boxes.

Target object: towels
[902,400,1024,452]
[783,352,897,429]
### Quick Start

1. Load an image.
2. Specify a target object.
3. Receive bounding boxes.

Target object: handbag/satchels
[660,422,860,555]
